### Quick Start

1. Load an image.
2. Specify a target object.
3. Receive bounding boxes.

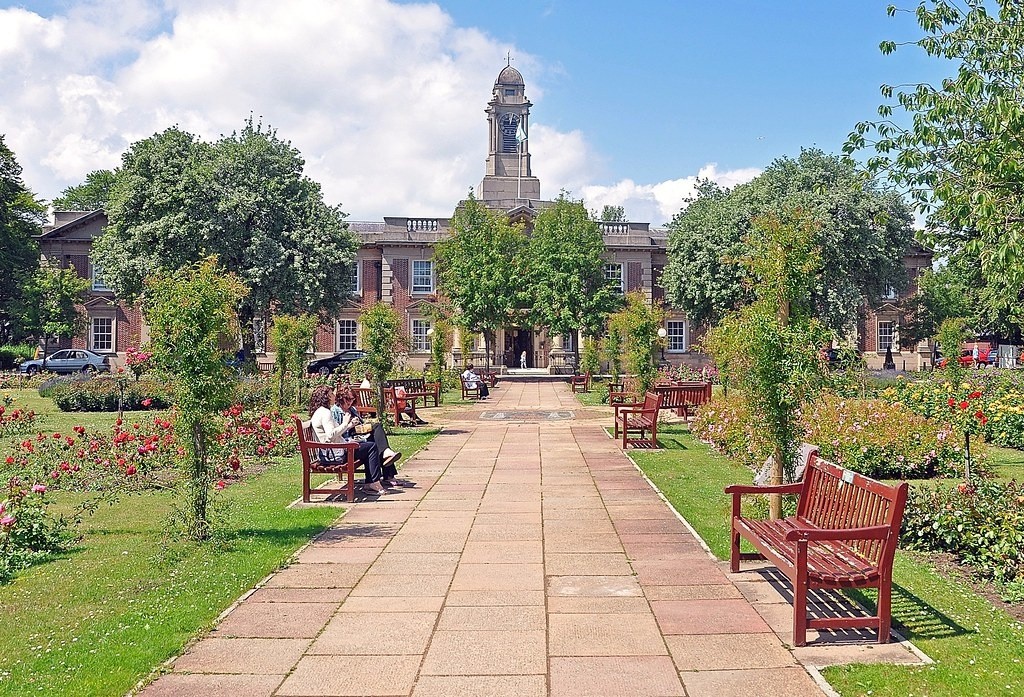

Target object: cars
[935,349,989,370]
[307,349,371,379]
[152,349,256,378]
[19,349,111,376]
[821,348,867,374]
[987,347,1024,365]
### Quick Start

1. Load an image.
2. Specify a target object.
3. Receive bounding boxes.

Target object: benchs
[651,381,712,420]
[460,374,481,399]
[386,378,440,408]
[571,371,589,393]
[723,449,910,648]
[258,363,277,373]
[337,384,418,426]
[480,369,497,388]
[296,417,367,503]
[611,392,663,450]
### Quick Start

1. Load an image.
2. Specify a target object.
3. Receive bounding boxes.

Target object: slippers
[384,452,402,467]
[363,489,389,495]
[385,477,417,487]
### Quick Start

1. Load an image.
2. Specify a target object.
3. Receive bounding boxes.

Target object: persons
[520,351,529,370]
[972,345,980,369]
[13,354,26,364]
[382,382,428,426]
[310,384,391,495]
[359,373,379,417]
[463,364,491,399]
[330,385,404,489]
